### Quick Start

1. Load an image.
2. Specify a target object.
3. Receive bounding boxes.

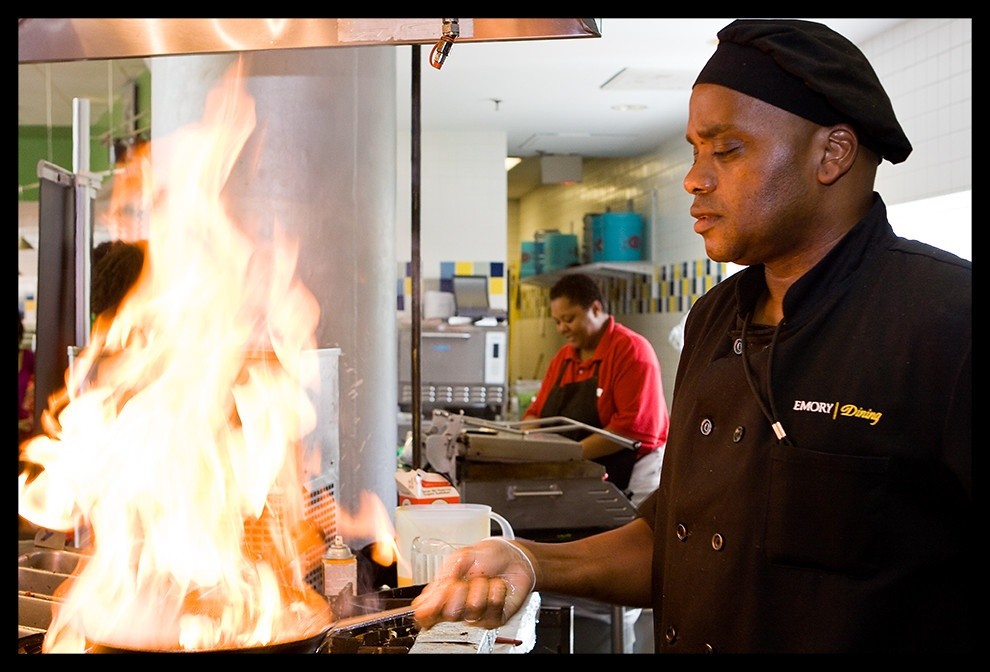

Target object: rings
[464,617,482,623]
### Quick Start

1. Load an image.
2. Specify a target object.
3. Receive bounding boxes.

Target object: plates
[425,291,455,320]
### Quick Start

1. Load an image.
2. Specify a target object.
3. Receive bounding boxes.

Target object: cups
[412,536,468,587]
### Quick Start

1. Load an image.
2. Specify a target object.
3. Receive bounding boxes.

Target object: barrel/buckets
[519,211,647,277]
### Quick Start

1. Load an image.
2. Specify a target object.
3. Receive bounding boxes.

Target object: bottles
[321,536,357,597]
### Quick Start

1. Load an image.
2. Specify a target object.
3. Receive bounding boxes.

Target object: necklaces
[742,311,793,446]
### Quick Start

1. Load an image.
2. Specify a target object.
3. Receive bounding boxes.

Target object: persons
[520,274,668,653]
[64,239,145,548]
[410,18,971,654]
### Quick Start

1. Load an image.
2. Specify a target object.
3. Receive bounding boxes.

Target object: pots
[82,604,416,654]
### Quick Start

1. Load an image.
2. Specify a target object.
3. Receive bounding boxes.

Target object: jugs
[394,502,515,590]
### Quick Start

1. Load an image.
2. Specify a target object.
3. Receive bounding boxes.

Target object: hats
[692,20,912,164]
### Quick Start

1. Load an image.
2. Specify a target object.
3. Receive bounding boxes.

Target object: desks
[463,479,638,654]
